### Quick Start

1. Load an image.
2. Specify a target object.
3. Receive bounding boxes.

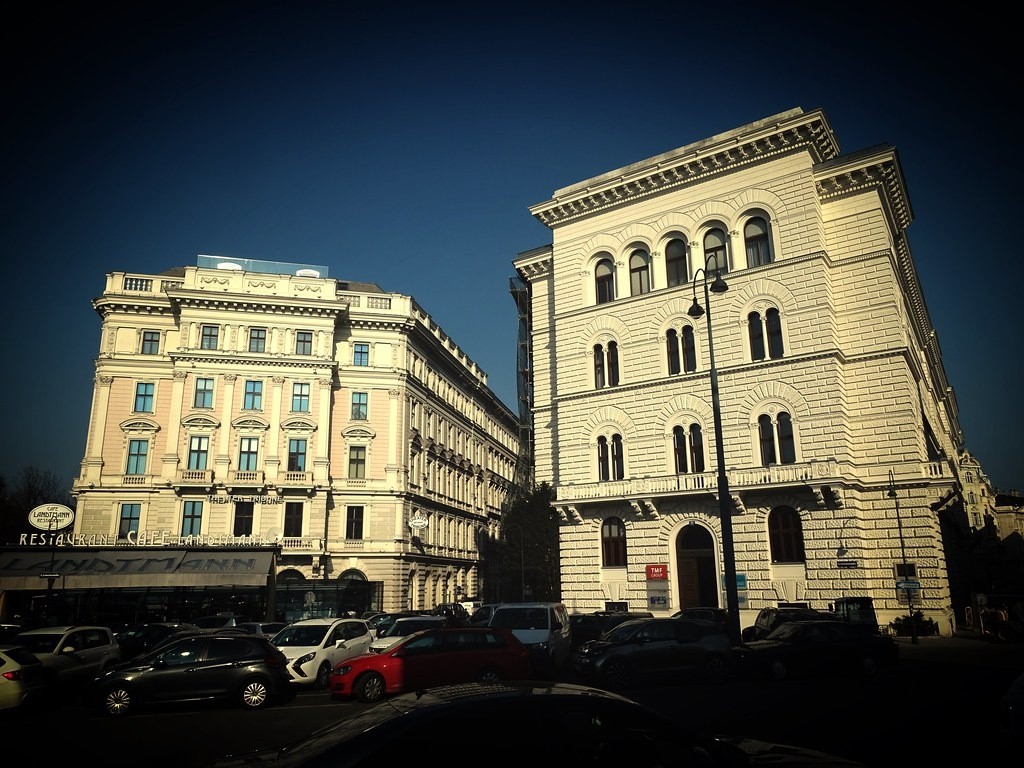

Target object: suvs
[9,625,119,696]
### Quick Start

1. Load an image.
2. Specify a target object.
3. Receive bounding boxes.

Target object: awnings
[0,549,274,592]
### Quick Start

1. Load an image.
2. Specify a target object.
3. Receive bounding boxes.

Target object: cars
[738,620,893,688]
[577,618,755,685]
[272,618,373,688]
[214,679,866,768]
[108,611,287,658]
[361,611,448,656]
[0,645,50,713]
[670,607,729,630]
[755,602,836,625]
[434,602,497,627]
[78,627,290,717]
[327,627,540,703]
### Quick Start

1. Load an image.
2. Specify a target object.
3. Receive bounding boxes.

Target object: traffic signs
[896,581,920,589]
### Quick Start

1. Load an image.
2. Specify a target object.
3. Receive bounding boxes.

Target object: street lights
[688,254,745,642]
[501,522,525,602]
[888,470,919,644]
[42,515,57,629]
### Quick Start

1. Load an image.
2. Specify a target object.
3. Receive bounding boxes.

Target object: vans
[489,602,570,669]
[569,612,653,644]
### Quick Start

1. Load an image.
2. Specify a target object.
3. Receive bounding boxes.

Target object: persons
[997,602,1009,641]
